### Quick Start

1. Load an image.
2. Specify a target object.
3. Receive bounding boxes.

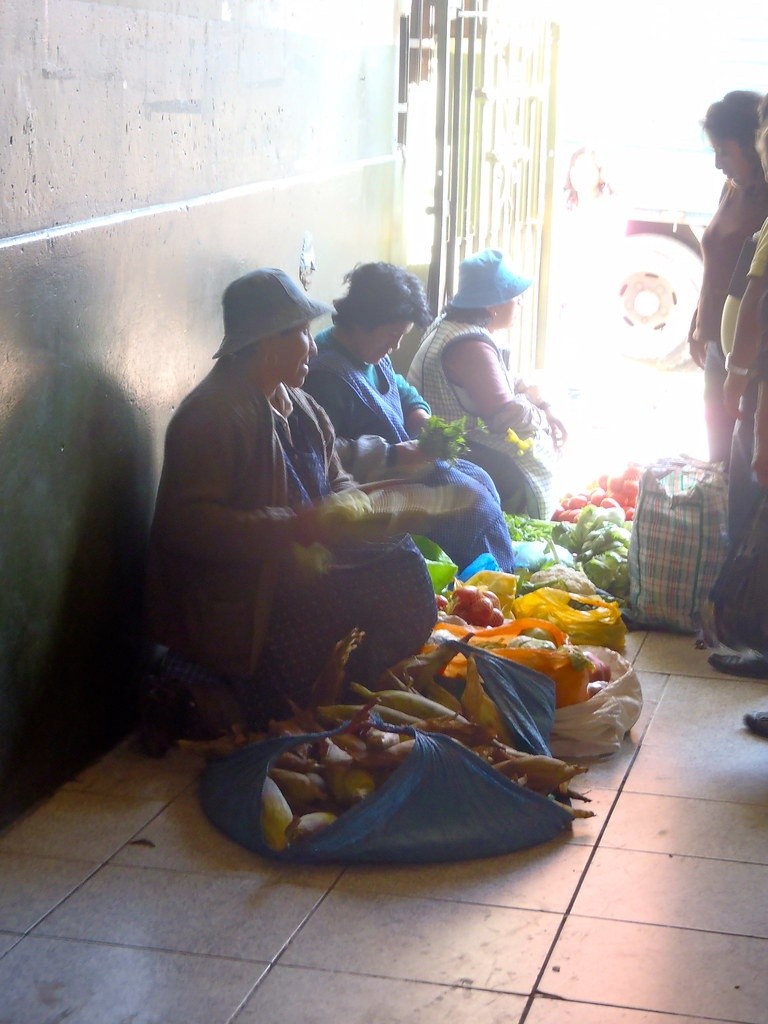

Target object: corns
[170,631,594,854]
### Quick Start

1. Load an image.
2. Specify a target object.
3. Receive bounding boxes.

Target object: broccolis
[527,563,596,597]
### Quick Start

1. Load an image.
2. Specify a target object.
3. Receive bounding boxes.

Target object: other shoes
[744,712,768,737]
[709,653,768,680]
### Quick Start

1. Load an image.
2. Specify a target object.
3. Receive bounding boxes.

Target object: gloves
[316,489,373,531]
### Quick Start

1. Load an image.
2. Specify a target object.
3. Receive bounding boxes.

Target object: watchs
[725,352,749,375]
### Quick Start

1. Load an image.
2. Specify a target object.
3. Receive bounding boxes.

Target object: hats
[451,249,532,309]
[212,268,335,359]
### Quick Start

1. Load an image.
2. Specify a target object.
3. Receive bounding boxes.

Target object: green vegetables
[415,415,488,464]
[500,511,553,543]
[553,507,631,607]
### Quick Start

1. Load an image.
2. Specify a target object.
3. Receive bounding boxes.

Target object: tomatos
[549,465,642,522]
[434,585,504,628]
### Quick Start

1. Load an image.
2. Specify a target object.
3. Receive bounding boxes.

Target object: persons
[687,92,768,735]
[147,248,566,760]
[566,150,612,207]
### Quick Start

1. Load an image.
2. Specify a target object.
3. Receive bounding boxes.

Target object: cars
[585,201,717,383]
